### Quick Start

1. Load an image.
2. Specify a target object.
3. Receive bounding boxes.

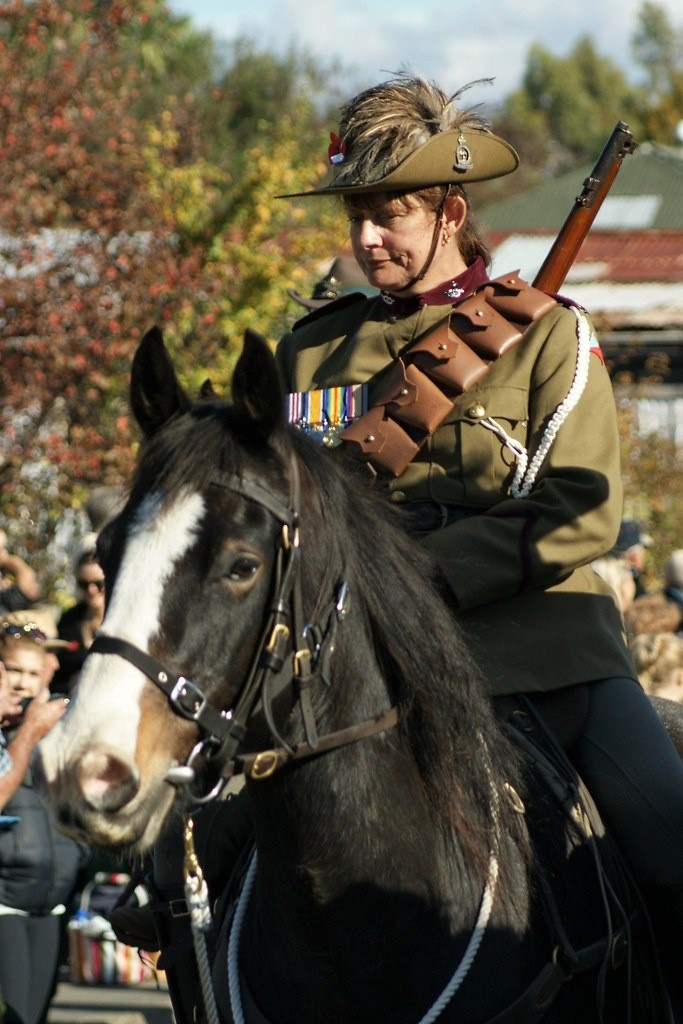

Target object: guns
[530,119,639,307]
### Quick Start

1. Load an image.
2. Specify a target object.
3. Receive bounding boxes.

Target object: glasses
[76,578,105,590]
[0,623,47,647]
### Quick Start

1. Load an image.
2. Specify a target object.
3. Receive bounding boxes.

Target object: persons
[274,73,683,1023]
[1,517,683,1024]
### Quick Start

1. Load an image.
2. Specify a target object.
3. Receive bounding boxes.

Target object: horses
[57,327,683,1024]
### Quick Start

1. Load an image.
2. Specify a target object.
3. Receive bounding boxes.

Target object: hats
[274,63,520,199]
[615,522,655,553]
